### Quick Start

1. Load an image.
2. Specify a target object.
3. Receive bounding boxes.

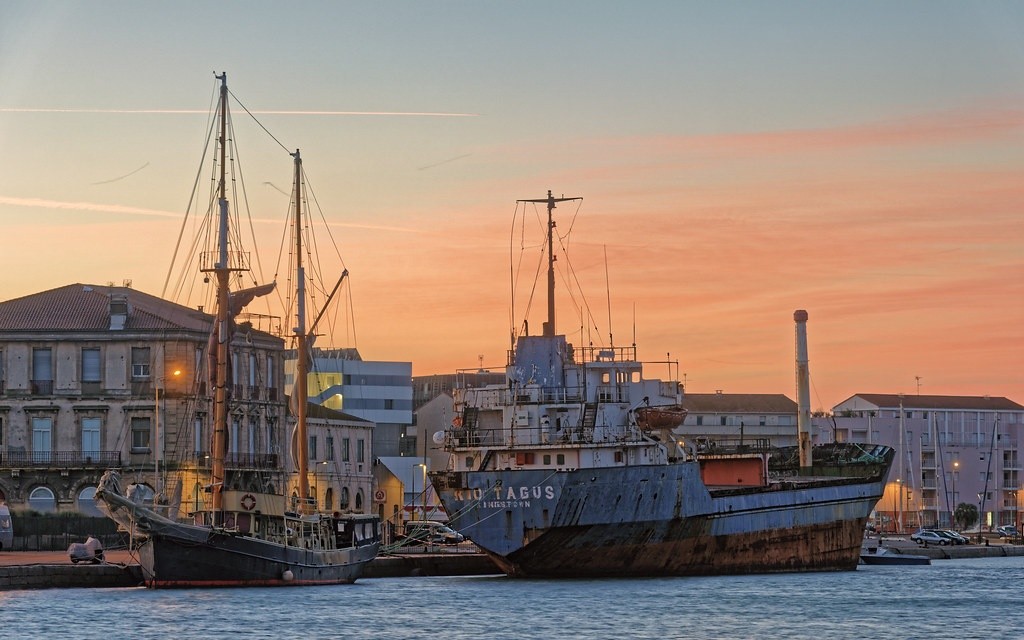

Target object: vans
[1002,526,1018,536]
[406,521,465,545]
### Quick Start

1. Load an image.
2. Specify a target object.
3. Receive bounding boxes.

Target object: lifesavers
[240,494,256,511]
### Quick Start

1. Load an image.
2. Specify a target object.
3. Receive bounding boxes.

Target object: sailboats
[96,71,378,588]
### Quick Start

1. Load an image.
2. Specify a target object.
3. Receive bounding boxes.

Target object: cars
[997,527,1007,536]
[945,530,969,544]
[922,528,965,546]
[910,531,951,546]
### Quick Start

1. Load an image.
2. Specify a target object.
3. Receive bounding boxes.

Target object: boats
[860,546,931,567]
[430,193,897,582]
